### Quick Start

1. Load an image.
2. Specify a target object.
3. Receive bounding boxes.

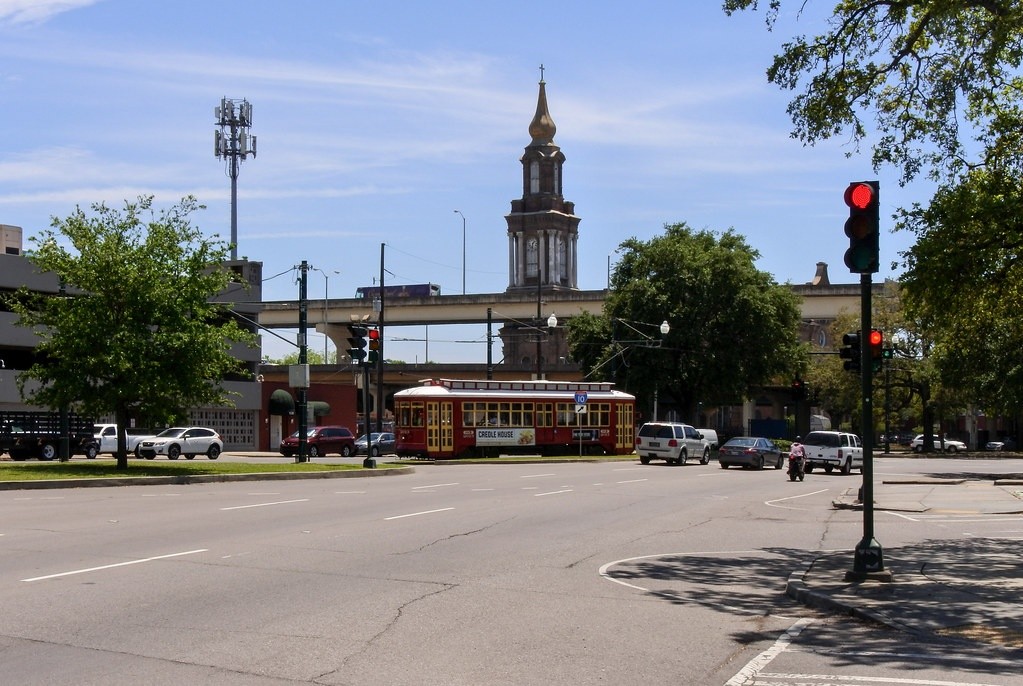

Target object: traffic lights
[369,330,379,361]
[791,379,804,401]
[844,181,880,269]
[839,333,860,374]
[863,329,883,372]
[346,326,367,360]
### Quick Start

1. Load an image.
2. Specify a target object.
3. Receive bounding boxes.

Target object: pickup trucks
[76,423,156,459]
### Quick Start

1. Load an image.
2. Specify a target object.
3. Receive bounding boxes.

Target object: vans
[695,428,719,451]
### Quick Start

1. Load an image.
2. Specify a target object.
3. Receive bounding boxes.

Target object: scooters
[787,452,811,481]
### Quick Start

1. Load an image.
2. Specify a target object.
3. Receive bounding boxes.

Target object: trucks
[0,410,95,459]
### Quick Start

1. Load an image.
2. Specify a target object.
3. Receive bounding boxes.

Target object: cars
[880,430,901,443]
[279,426,355,457]
[717,436,784,471]
[985,436,1018,452]
[355,432,396,456]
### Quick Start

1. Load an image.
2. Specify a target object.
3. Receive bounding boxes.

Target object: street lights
[313,268,328,364]
[611,313,670,381]
[486,307,557,380]
[372,296,385,432]
[454,209,465,295]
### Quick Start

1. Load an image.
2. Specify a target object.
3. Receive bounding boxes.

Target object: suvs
[138,426,224,460]
[635,421,711,466]
[802,430,864,476]
[910,434,967,454]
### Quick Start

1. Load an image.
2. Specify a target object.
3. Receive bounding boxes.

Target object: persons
[790,436,806,477]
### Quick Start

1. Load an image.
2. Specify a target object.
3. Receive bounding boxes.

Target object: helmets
[794,436,802,442]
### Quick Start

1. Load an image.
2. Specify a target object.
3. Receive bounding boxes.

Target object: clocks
[528,238,538,249]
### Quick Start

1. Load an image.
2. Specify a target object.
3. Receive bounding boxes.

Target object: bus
[393,378,637,462]
